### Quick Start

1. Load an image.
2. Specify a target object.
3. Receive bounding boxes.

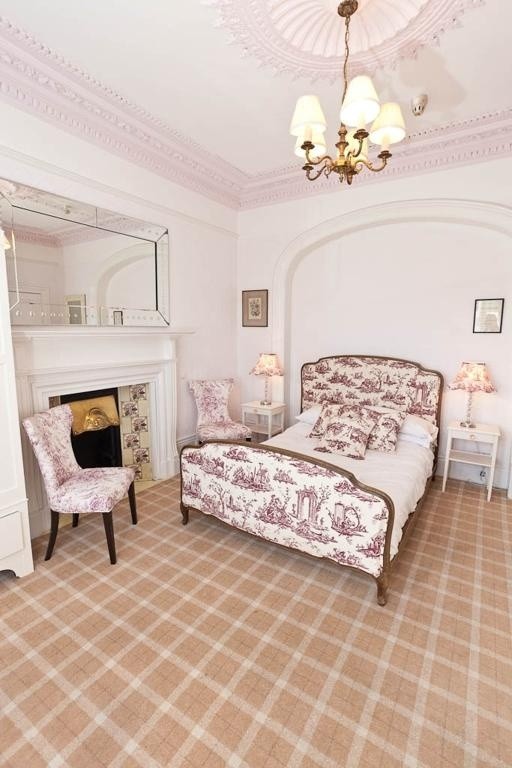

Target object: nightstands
[241,401,286,440]
[442,420,500,502]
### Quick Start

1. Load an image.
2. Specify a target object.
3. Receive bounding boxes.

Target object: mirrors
[0,177,170,328]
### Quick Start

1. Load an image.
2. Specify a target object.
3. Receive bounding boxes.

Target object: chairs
[189,378,253,447]
[23,404,139,564]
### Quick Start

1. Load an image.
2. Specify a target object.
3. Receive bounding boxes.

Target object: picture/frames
[113,310,123,324]
[242,289,268,327]
[473,298,504,333]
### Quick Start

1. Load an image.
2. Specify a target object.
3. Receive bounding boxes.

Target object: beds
[179,354,444,606]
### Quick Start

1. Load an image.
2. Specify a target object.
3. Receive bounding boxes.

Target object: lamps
[447,362,498,429]
[249,354,285,405]
[289,0,407,185]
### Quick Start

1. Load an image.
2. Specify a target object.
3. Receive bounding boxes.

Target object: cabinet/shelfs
[0,227,35,578]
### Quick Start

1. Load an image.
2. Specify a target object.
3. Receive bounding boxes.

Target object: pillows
[397,433,437,448]
[295,405,322,426]
[314,404,382,461]
[306,403,408,454]
[364,404,436,441]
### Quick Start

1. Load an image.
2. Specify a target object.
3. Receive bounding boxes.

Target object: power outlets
[480,471,486,477]
